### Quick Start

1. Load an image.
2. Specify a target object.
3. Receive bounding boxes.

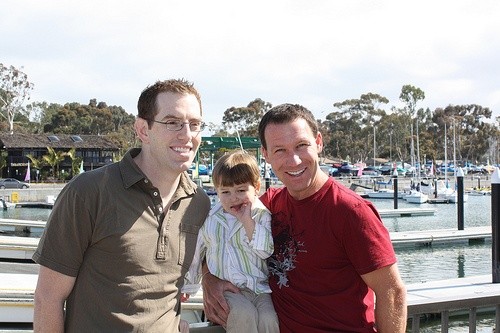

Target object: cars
[0,178,30,189]
[331,162,497,176]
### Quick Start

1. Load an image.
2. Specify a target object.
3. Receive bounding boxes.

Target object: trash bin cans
[11,192,18,203]
[193,178,204,189]
[352,169,359,176]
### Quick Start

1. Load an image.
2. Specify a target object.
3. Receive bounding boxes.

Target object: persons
[204,102,409,333]
[181,152,279,333]
[33,80,213,333]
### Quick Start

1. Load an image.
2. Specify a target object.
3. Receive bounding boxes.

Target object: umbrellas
[392,160,398,176]
[357,163,363,178]
[429,159,437,182]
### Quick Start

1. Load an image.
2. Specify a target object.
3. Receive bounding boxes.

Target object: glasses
[143,117,208,132]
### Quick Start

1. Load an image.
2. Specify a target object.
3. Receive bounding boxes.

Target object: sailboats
[366,119,469,204]
